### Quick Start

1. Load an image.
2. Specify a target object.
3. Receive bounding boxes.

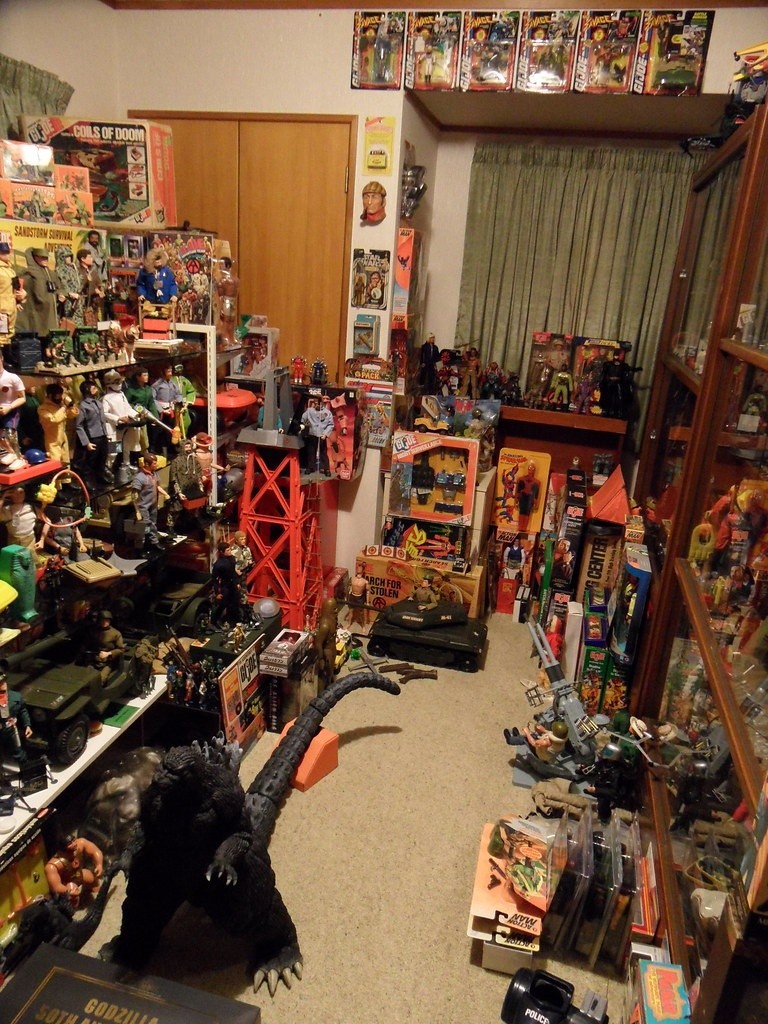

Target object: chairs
[105,654,124,686]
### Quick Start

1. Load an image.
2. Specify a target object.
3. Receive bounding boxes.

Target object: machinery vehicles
[110,568,216,638]
[366,598,488,673]
[0,619,159,765]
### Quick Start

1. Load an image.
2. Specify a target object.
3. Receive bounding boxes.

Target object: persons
[291,356,329,385]
[90,610,124,684]
[0,350,26,466]
[137,255,179,305]
[515,464,541,532]
[0,672,32,764]
[210,531,255,631]
[620,717,647,760]
[408,575,438,610]
[0,230,110,350]
[0,486,89,567]
[301,398,334,476]
[166,653,223,709]
[44,831,104,908]
[131,453,171,551]
[503,539,526,570]
[504,722,569,762]
[258,407,284,434]
[418,332,643,420]
[316,564,371,693]
[167,432,230,534]
[659,723,680,764]
[363,181,387,222]
[24,361,195,493]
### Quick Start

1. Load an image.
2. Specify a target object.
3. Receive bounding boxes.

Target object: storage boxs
[229,326,279,379]
[495,469,587,631]
[575,528,622,602]
[189,612,282,668]
[392,228,423,331]
[578,646,631,717]
[258,627,320,733]
[224,378,368,482]
[391,329,416,396]
[0,116,178,229]
[324,568,349,606]
[355,516,487,625]
[619,856,693,1024]
[0,218,218,335]
[141,694,223,748]
[482,941,533,974]
[560,601,583,684]
[624,513,646,545]
[525,331,632,401]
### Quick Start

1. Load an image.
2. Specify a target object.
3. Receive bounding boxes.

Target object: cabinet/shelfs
[493,405,628,495]
[383,467,497,565]
[0,322,260,849]
[635,101,768,1024]
